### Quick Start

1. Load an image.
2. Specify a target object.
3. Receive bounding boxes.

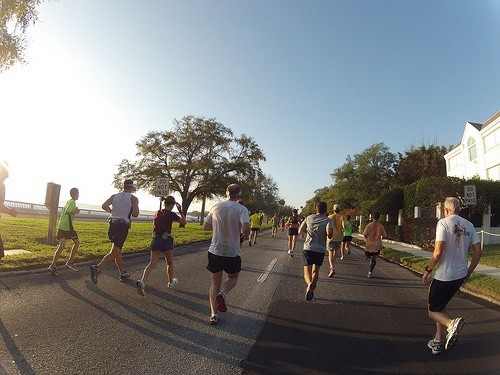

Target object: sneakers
[427,339,442,355]
[445,318,466,350]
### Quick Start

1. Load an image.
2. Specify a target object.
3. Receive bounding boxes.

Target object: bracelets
[179,210,182,213]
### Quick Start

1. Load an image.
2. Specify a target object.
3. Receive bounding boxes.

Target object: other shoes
[136,279,146,296]
[304,282,316,302]
[253,241,257,244]
[367,272,372,278]
[216,292,228,312]
[340,256,344,260]
[288,249,295,257]
[90,264,101,284]
[167,277,179,289]
[347,246,351,256]
[328,268,335,277]
[47,264,59,276]
[119,271,130,282]
[64,261,80,272]
[207,313,221,324]
[248,241,251,246]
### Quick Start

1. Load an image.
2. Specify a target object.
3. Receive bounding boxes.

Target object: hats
[334,204,341,211]
[124,179,133,184]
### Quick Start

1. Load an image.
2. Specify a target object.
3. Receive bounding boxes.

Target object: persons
[90,179,139,285]
[135,196,186,297]
[285,209,302,258]
[298,201,334,301]
[326,204,346,278]
[249,209,262,246]
[340,216,353,260]
[422,197,482,355]
[203,183,250,325]
[363,211,387,278]
[46,188,80,276]
[271,213,307,237]
[0,165,18,259]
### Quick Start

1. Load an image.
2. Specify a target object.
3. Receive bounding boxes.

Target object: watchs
[425,265,432,273]
